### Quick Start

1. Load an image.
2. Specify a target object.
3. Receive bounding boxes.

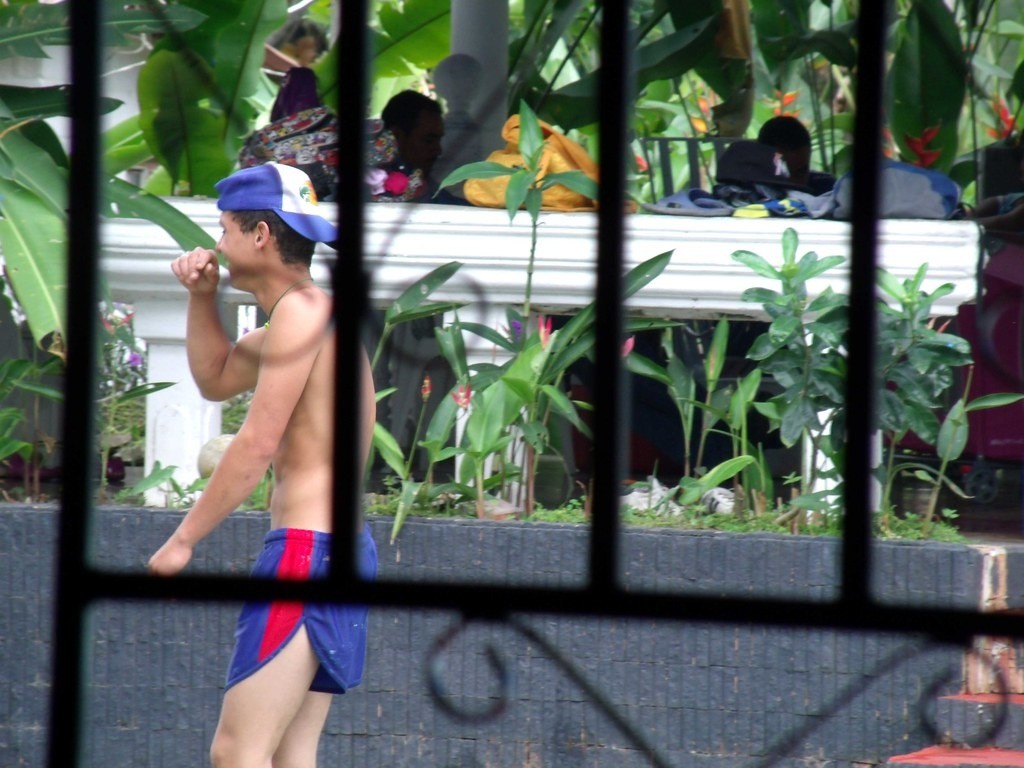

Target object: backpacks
[238,106,397,196]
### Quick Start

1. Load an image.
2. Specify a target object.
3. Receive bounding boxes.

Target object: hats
[716,139,808,191]
[214,161,338,243]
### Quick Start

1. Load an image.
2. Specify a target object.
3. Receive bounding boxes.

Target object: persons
[267,65,324,121]
[717,117,838,217]
[147,161,378,768]
[380,88,467,205]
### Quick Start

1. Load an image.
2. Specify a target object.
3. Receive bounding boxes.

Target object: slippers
[642,188,732,216]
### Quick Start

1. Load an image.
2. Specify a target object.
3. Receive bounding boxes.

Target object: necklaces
[264,278,314,327]
